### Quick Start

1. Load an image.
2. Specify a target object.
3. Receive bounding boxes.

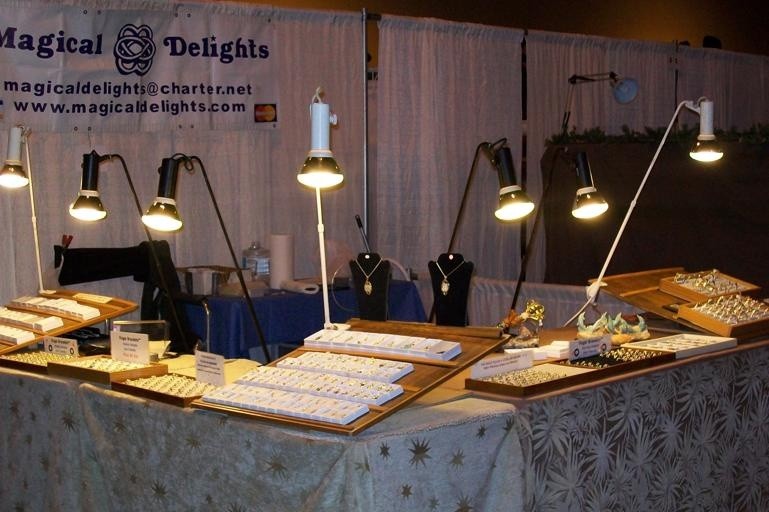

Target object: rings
[559,347,663,369]
[474,367,567,389]
[0,350,218,399]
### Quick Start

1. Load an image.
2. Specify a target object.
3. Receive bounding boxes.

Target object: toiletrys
[280,280,320,295]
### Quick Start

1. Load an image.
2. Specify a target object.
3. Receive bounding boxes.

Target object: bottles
[241,240,269,283]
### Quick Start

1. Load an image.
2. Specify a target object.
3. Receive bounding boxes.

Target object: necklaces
[435,260,466,297]
[353,258,383,296]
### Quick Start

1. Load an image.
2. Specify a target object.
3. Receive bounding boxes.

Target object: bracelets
[670,267,769,325]
